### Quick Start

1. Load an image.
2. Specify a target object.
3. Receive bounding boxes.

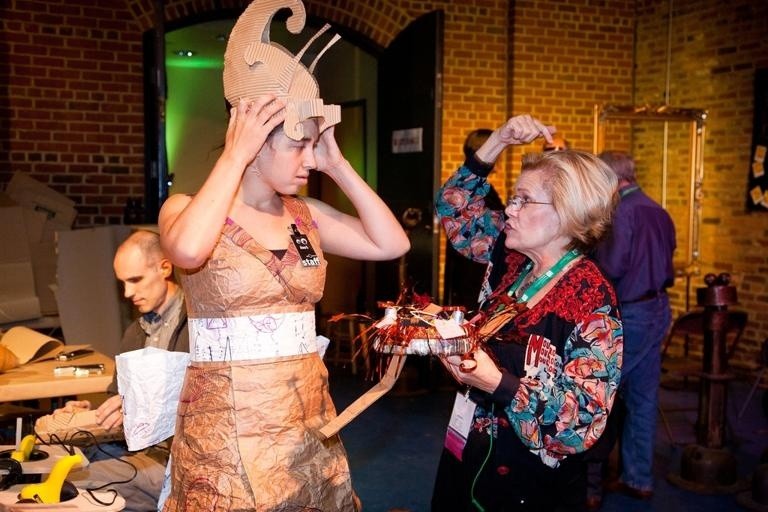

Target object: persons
[441,125,508,308]
[151,85,413,511]
[51,230,191,512]
[586,147,678,511]
[427,114,626,511]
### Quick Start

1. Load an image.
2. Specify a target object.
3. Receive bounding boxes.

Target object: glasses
[506,195,554,211]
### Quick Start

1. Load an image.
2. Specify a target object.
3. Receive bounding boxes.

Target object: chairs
[661,311,746,444]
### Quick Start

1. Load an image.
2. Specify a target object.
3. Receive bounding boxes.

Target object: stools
[324,311,373,374]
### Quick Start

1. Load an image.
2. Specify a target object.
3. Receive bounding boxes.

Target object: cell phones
[62,348,94,361]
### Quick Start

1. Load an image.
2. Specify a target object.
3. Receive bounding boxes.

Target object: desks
[0,346,118,410]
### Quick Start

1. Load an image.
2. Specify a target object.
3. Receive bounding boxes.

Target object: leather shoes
[608,477,653,501]
[587,494,602,511]
